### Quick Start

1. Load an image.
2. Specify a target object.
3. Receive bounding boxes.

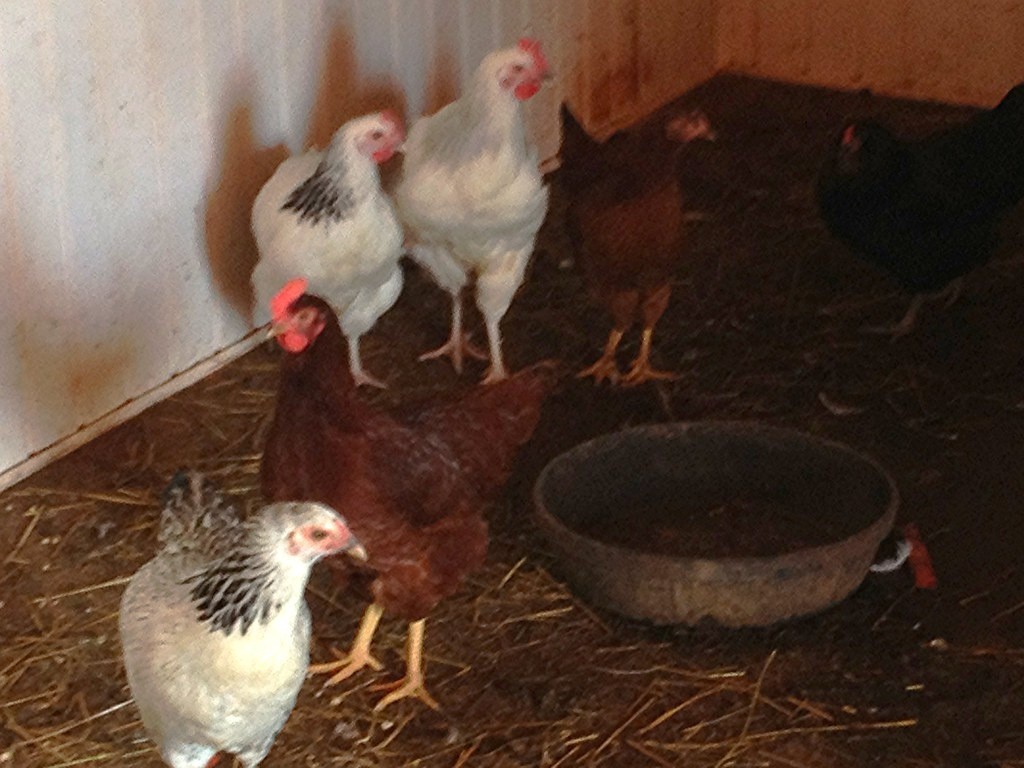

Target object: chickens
[118,37,1024,768]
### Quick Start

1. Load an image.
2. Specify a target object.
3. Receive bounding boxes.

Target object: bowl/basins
[535,421,897,633]
